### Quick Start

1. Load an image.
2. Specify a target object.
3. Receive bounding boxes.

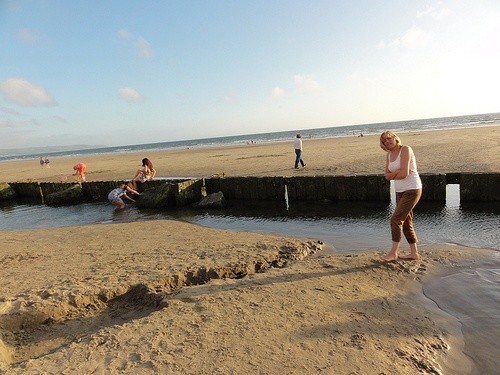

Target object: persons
[133,158,156,182]
[45,157,50,168]
[379,131,423,261]
[40,157,44,166]
[108,183,143,209]
[293,134,306,169]
[73,162,86,179]
